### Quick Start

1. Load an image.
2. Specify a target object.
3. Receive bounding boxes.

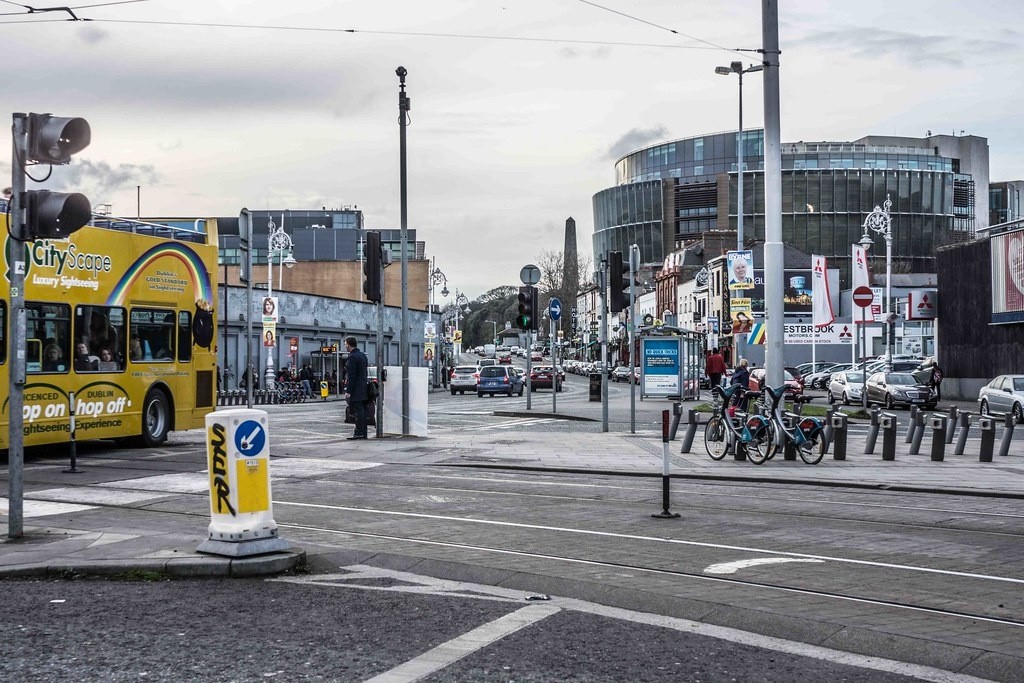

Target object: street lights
[454,292,471,364]
[715,60,764,364]
[266,214,301,389]
[691,260,715,356]
[427,256,449,393]
[485,319,511,366]
[858,191,896,373]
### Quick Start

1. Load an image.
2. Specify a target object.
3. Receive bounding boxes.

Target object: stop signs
[853,286,874,307]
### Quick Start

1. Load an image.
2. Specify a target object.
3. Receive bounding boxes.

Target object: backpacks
[934,368,942,383]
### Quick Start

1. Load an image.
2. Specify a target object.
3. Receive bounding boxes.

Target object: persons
[730,258,753,285]
[705,348,728,401]
[930,362,944,400]
[241,367,259,391]
[217,365,222,398]
[263,297,276,316]
[264,331,276,345]
[47,335,143,372]
[708,322,718,334]
[341,336,371,441]
[426,325,435,336]
[440,353,445,364]
[277,368,290,383]
[326,369,337,393]
[454,334,461,341]
[441,365,454,389]
[730,359,750,411]
[300,363,315,399]
[733,312,753,332]
[424,349,433,360]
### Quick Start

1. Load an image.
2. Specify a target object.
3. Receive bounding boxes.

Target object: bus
[0,190,221,458]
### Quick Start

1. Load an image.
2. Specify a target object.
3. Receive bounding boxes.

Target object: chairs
[99,324,183,371]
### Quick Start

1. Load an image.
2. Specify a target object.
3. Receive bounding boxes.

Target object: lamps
[314,319,319,327]
[366,324,370,330]
[341,322,346,328]
[280,316,286,324]
[239,314,245,321]
[389,327,393,332]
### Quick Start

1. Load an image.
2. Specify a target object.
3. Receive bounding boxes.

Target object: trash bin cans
[589,373,602,402]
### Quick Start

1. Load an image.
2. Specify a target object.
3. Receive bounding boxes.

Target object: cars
[465,337,552,387]
[796,354,935,391]
[977,374,1024,424]
[700,364,762,392]
[748,366,803,401]
[827,370,873,406]
[860,372,939,411]
[551,358,642,386]
[682,368,699,396]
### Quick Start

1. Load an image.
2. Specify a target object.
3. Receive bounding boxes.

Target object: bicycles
[272,380,308,404]
[755,382,826,465]
[704,383,772,466]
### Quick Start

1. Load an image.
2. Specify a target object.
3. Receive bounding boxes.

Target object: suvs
[449,364,483,396]
[477,365,524,398]
[530,365,563,392]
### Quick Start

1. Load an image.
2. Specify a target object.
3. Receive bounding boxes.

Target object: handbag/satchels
[239,379,246,388]
[365,381,379,405]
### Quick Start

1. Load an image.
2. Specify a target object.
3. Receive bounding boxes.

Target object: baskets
[755,392,785,411]
[703,391,734,410]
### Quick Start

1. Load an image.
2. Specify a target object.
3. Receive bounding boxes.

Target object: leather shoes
[345,434,367,440]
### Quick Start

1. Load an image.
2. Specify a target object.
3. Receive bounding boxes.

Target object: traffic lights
[362,243,370,296]
[28,114,92,165]
[26,188,94,239]
[496,340,501,346]
[516,292,532,330]
[620,259,639,310]
